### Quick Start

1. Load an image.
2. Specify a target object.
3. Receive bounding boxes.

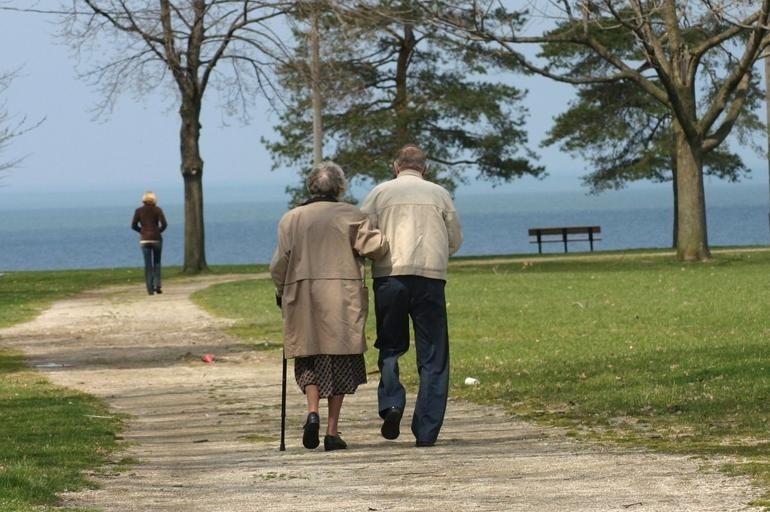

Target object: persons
[130,192,168,295]
[361,143,465,446]
[269,158,389,456]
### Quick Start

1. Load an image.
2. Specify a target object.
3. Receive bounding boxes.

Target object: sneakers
[381,405,402,440]
[303,411,320,451]
[324,431,347,451]
[414,435,438,447]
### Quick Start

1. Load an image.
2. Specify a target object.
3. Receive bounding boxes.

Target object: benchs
[527,224,602,253]
